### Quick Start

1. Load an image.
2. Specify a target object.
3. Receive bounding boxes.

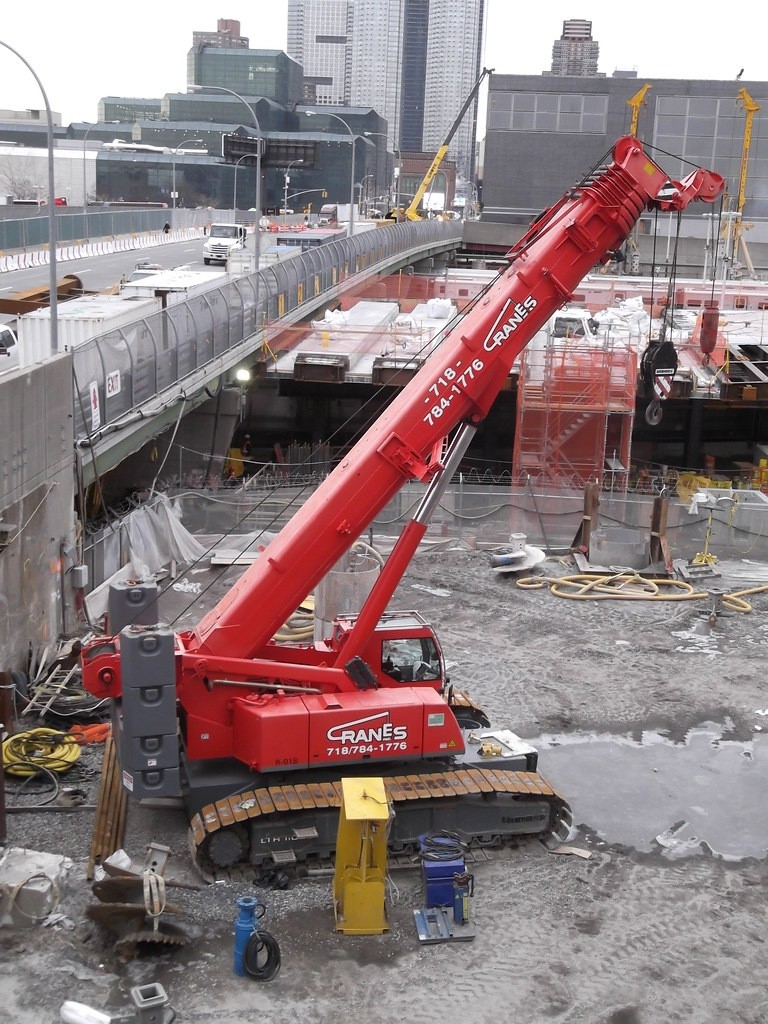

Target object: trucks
[203,223,247,267]
[319,204,357,229]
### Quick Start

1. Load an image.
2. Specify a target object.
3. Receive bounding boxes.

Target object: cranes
[78,138,725,884]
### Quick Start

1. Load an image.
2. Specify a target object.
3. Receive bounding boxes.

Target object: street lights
[234,152,257,225]
[467,180,477,220]
[186,84,261,267]
[306,110,355,234]
[284,159,304,214]
[358,174,374,212]
[82,121,121,247]
[364,131,400,223]
[172,138,205,223]
